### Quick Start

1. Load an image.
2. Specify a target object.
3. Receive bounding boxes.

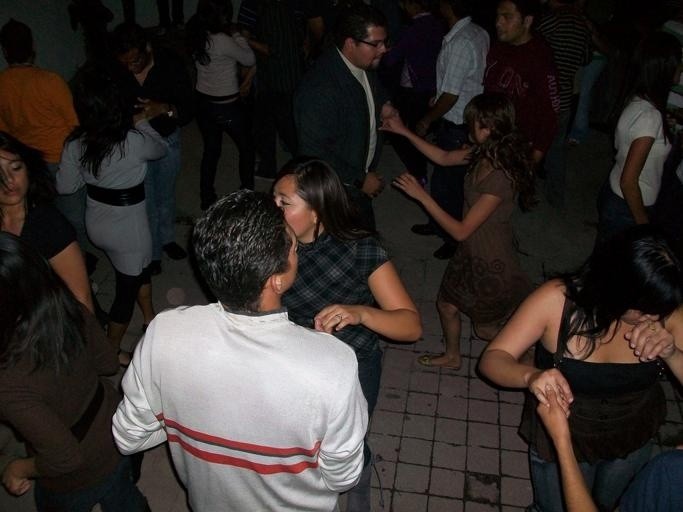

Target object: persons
[0,0,683,512]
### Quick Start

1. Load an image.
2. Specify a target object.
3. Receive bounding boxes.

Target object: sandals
[117,349,133,367]
[417,355,462,370]
[142,325,149,332]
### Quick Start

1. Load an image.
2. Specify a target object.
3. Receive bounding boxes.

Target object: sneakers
[434,242,461,259]
[411,224,440,235]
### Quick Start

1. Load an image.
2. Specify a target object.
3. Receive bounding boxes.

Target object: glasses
[361,39,391,48]
